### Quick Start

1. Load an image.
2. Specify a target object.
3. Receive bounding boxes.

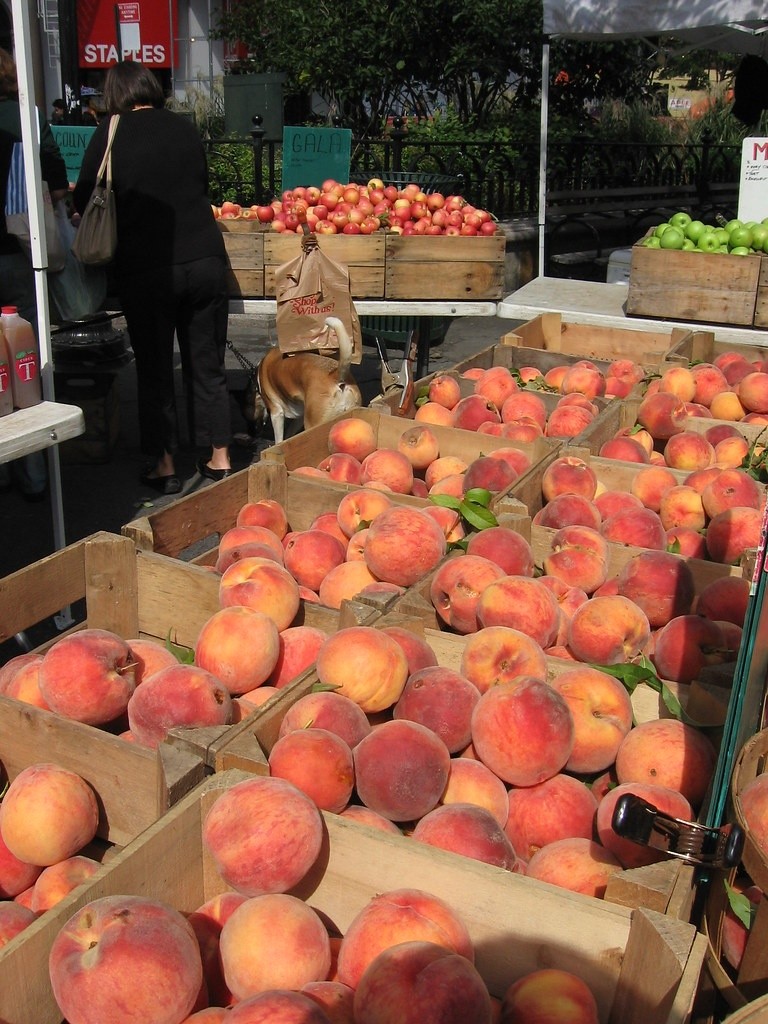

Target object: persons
[68,59,231,500]
[50,97,99,126]
[0,47,70,504]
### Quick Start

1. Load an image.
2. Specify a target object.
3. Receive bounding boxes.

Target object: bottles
[0,304,40,418]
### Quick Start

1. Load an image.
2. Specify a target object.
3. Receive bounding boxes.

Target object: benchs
[536,183,739,281]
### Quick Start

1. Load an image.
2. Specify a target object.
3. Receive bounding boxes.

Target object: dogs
[242,316,362,444]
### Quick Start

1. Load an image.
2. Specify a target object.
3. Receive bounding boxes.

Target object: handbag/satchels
[4,142,107,319]
[70,114,120,267]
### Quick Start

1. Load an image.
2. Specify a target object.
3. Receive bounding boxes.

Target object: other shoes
[138,467,182,493]
[195,455,233,482]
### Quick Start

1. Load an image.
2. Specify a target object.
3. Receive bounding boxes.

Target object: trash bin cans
[356,316,459,345]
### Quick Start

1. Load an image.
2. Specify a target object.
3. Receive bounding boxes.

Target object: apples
[210,178,498,237]
[643,211,768,256]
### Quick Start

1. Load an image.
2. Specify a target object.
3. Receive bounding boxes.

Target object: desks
[496,277,768,348]
[0,397,83,465]
[228,298,497,383]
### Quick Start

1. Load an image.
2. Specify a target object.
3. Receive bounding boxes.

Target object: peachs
[210,416,767,685]
[414,351,768,479]
[0,553,768,1024]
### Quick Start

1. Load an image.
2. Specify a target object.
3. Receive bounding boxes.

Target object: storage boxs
[0,219,768,1024]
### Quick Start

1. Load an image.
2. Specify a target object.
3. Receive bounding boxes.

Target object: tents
[537,0,768,284]
